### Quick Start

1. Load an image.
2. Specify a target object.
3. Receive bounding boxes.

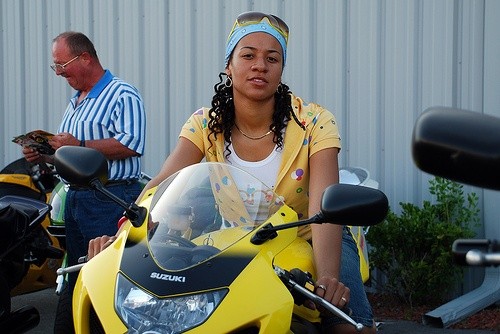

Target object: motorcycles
[0,194,52,334]
[52,145,390,333]
[411,103,499,269]
[0,157,153,295]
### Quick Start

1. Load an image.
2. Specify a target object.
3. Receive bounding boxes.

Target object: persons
[22,32,146,334]
[87,11,377,334]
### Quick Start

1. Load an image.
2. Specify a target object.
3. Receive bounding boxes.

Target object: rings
[318,284,326,290]
[342,296,347,302]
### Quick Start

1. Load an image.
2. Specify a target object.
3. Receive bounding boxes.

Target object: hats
[224,19,288,68]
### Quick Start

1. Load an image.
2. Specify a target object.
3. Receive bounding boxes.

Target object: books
[11,128,57,156]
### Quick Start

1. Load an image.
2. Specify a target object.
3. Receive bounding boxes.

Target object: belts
[69,178,138,190]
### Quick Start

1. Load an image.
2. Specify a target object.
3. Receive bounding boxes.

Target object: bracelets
[79,139,85,147]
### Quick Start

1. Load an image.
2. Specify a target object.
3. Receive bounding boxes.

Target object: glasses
[226,10,289,49]
[50,54,81,73]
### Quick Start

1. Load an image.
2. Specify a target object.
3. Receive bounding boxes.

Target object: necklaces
[234,123,275,140]
